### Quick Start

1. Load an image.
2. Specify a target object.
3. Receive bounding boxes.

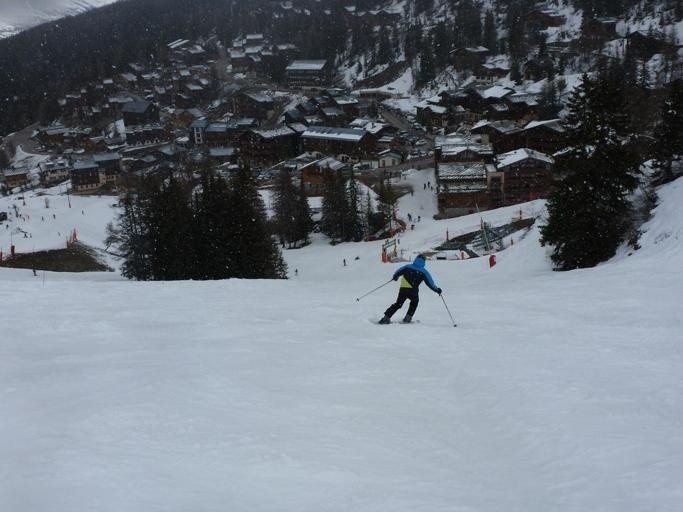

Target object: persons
[342,181,434,267]
[375,255,442,325]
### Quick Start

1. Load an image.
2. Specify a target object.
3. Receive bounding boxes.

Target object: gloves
[393,274,398,281]
[435,288,442,294]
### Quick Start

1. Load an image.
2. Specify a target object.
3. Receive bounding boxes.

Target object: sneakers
[404,316,411,322]
[380,318,390,324]
